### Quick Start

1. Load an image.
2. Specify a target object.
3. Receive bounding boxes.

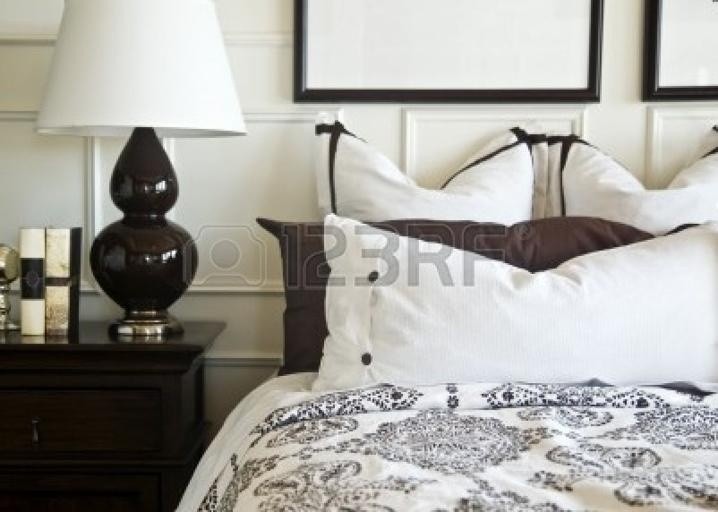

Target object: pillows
[547,124,718,237]
[307,215,718,395]
[255,215,701,374]
[314,110,548,226]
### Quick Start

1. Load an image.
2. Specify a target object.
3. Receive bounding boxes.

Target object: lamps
[34,0,248,338]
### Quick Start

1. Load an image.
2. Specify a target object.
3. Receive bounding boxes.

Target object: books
[17,228,43,335]
[46,226,83,337]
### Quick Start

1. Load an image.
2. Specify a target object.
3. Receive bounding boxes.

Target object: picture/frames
[294,0,602,105]
[643,0,718,103]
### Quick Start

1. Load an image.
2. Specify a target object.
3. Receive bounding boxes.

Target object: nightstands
[0,317,226,512]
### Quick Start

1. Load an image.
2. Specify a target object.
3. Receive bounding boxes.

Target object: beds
[172,371,718,512]
